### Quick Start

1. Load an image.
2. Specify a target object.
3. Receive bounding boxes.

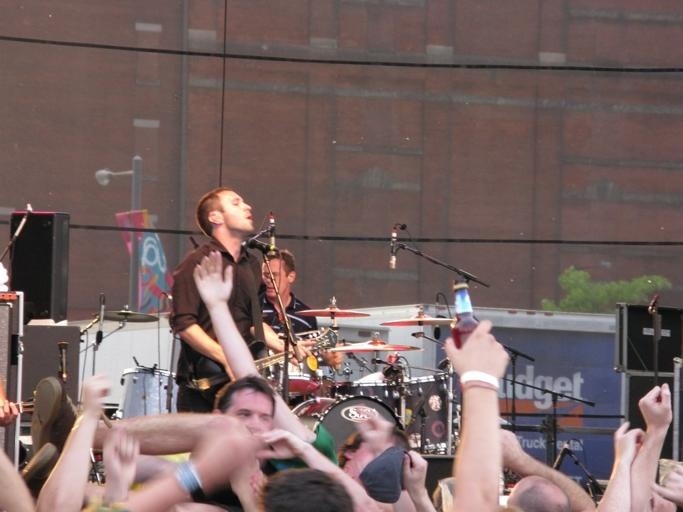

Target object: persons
[1,249,509,511]
[500,382,683,511]
[164,185,317,414]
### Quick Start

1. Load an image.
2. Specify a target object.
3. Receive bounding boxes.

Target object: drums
[116,368,179,418]
[292,395,404,458]
[351,373,451,454]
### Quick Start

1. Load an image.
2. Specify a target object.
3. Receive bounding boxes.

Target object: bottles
[450,278,481,349]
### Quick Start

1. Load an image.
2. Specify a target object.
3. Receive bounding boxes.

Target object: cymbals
[92,311,160,322]
[329,341,422,351]
[295,308,370,316]
[380,314,454,326]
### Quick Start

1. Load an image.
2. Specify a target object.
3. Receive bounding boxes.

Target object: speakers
[9,210,69,324]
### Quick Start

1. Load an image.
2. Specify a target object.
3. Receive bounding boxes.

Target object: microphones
[647,292,660,315]
[269,218,278,253]
[247,236,275,254]
[433,301,441,340]
[390,229,397,269]
[95,291,103,345]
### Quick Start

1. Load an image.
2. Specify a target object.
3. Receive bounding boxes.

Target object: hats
[358,445,414,503]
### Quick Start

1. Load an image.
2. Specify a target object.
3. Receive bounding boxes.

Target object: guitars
[193,328,337,401]
